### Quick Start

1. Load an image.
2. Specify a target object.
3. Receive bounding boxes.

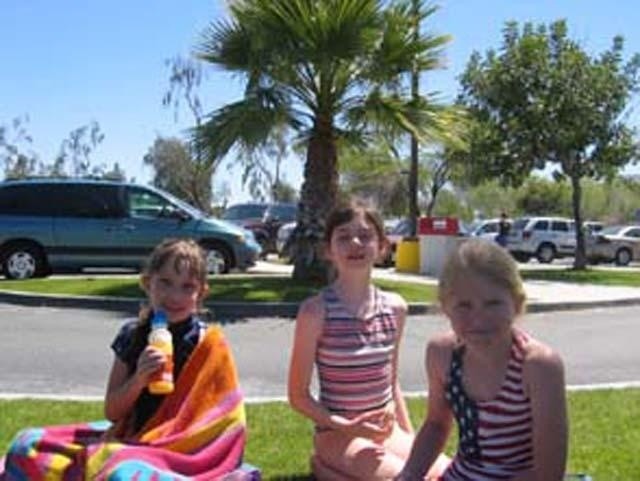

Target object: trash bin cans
[416,216,459,275]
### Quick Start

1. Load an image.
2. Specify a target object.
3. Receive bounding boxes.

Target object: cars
[220,203,297,262]
[377,216,640,266]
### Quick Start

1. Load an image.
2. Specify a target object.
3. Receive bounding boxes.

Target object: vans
[0,176,263,279]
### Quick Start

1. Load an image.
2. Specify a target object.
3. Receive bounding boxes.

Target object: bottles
[144,312,175,394]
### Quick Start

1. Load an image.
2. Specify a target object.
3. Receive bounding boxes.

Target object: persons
[1,235,260,481]
[393,237,568,481]
[494,212,511,249]
[286,195,453,481]
